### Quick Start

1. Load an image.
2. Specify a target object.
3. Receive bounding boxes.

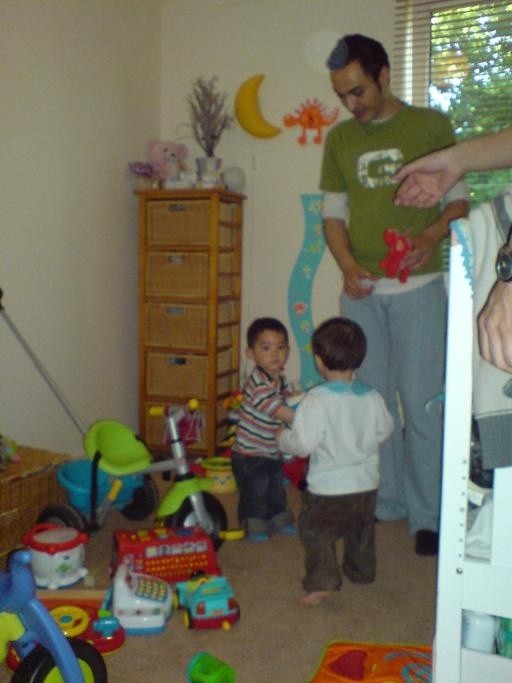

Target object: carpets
[307,641,432,683]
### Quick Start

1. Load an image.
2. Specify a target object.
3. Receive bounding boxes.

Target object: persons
[231,316,297,540]
[274,315,394,605]
[394,126,511,375]
[318,34,469,553]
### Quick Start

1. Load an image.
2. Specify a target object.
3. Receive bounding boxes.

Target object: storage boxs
[0,447,71,572]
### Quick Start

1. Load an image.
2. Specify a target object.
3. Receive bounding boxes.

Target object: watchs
[494,245,511,283]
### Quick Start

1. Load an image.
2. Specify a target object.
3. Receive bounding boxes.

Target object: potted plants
[173,75,237,189]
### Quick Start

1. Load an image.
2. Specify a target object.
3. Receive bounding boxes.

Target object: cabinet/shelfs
[135,189,247,458]
[431,217,512,682]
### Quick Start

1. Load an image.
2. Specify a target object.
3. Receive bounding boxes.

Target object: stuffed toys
[147,135,188,178]
